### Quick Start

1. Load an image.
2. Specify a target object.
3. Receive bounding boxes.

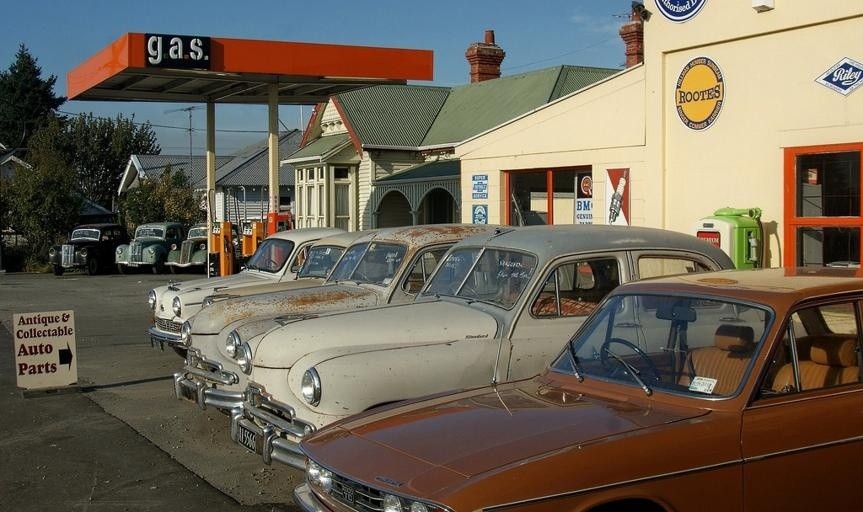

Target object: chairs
[674,324,861,392]
